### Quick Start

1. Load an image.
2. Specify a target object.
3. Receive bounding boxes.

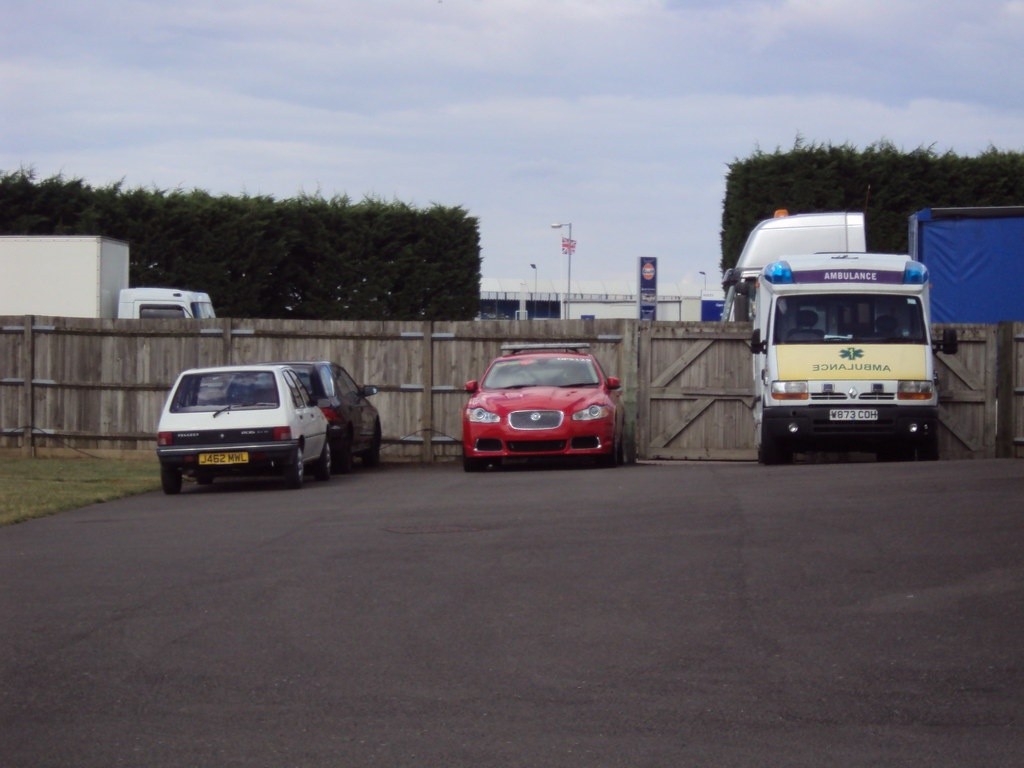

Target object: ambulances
[750,251,958,464]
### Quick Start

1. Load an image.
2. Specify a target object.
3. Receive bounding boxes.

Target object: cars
[222,361,382,473]
[460,339,627,472]
[153,364,334,495]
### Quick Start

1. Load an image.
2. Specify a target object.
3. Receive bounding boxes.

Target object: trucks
[0,233,216,320]
[719,203,1022,323]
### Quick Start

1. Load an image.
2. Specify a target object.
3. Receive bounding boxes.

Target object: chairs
[788,310,824,336]
[873,316,901,337]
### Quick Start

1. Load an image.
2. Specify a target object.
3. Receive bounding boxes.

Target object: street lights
[551,222,572,319]
[530,263,538,319]
[698,271,707,292]
